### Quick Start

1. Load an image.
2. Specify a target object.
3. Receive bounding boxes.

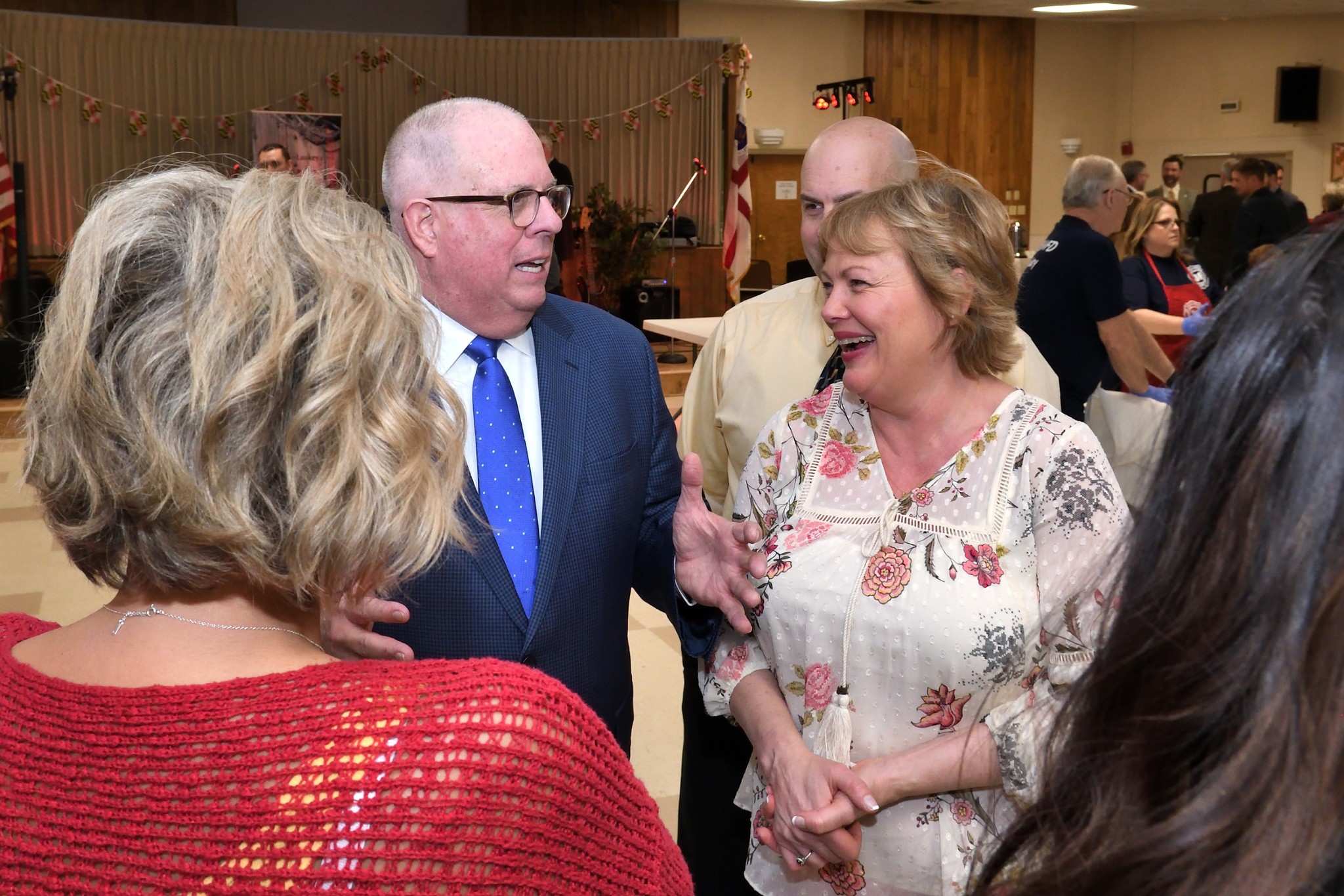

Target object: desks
[643,317,725,419]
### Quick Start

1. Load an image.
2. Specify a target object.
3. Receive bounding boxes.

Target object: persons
[1012,154,1178,425]
[1101,198,1217,398]
[1185,159,1344,307]
[315,98,770,765]
[0,159,699,896]
[1120,160,1150,232]
[258,143,293,174]
[533,128,574,298]
[702,150,1134,896]
[1146,155,1199,259]
[673,114,1064,896]
[959,225,1343,896]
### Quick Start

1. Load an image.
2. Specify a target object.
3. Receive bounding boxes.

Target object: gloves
[1183,303,1213,338]
[1130,384,1173,405]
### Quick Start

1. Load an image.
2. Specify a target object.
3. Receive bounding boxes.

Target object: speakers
[619,284,681,342]
[1273,65,1321,124]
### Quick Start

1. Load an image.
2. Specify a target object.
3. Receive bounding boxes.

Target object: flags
[0,133,17,281]
[722,77,752,304]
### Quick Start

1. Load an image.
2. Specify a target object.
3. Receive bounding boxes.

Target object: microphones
[693,157,708,176]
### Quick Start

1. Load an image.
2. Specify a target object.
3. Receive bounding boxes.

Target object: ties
[1168,189,1176,202]
[462,335,540,617]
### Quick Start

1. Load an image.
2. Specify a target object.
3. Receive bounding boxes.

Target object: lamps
[810,75,875,110]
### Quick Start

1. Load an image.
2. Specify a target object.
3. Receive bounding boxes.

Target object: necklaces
[104,600,327,654]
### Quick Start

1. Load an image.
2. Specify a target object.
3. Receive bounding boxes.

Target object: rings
[795,851,813,866]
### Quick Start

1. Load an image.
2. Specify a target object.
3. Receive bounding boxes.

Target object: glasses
[400,184,574,227]
[1149,219,1184,229]
[1103,188,1136,205]
